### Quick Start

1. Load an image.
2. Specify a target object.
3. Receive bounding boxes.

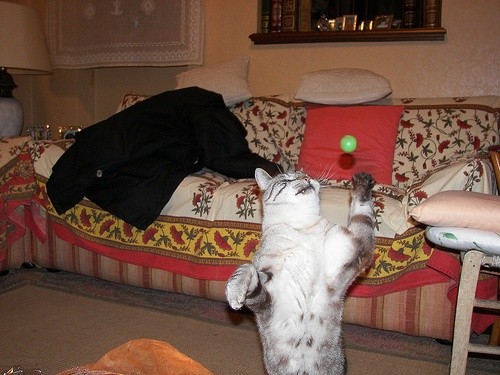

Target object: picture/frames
[360,20,374,30]
[372,14,394,31]
[342,14,358,30]
[391,19,401,29]
[335,17,343,31]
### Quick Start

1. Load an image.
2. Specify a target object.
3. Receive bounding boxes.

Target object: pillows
[174,55,253,107]
[408,190,500,233]
[294,68,393,105]
[425,225,500,256]
[296,104,405,186]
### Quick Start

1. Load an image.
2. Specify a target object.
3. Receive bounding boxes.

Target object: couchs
[0,52,500,348]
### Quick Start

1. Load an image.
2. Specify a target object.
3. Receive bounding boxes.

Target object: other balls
[340,135,357,152]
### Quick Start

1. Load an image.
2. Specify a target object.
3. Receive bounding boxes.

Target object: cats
[224,158,377,375]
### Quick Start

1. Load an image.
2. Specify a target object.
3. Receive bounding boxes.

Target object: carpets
[45,0,205,69]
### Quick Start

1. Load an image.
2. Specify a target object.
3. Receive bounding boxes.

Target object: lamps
[0,2,55,139]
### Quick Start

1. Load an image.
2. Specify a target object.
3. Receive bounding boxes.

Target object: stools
[449,250,500,375]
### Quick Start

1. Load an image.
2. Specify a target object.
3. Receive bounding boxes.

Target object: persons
[337,16,399,31]
